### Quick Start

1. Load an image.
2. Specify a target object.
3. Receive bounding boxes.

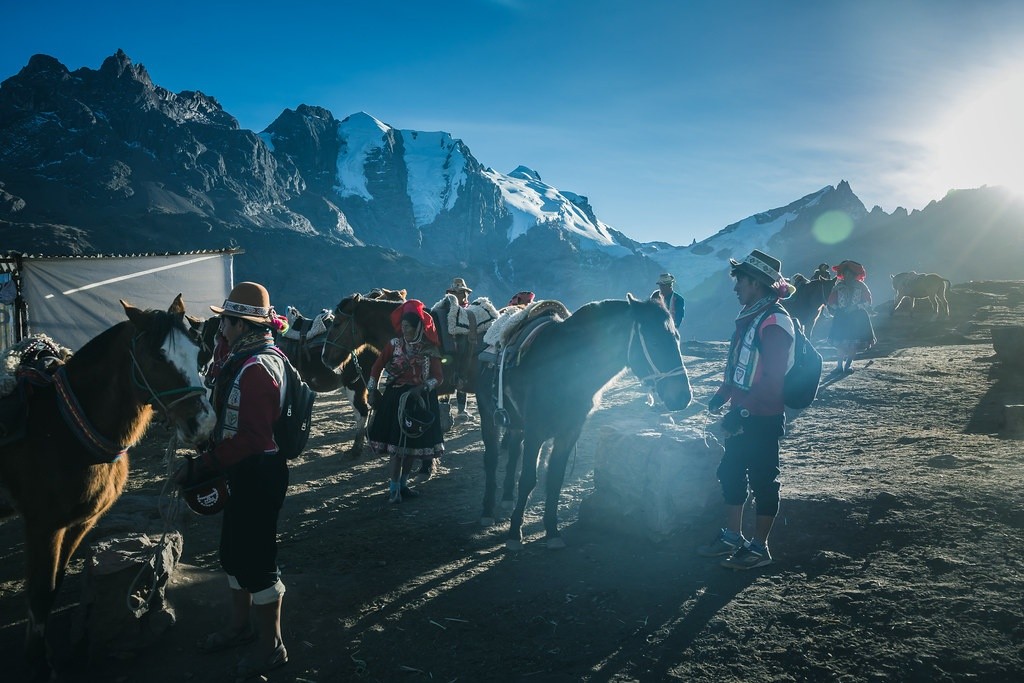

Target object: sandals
[225,642,289,683]
[193,625,257,653]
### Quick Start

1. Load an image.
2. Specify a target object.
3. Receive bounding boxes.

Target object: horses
[777,273,837,341]
[477,290,692,550]
[321,293,508,503]
[0,293,218,683]
[185,288,409,462]
[890,272,952,324]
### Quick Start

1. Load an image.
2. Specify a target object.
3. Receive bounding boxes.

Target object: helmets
[178,472,231,517]
[401,408,436,435]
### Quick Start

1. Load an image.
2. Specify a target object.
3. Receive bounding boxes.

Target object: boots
[844,358,854,373]
[830,358,843,374]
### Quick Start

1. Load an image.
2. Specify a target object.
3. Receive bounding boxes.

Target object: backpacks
[755,307,822,409]
[224,343,316,459]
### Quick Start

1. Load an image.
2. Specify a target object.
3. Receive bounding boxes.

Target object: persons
[649,273,685,330]
[364,299,446,504]
[173,281,290,681]
[446,278,472,309]
[697,249,796,570]
[811,263,831,281]
[439,390,474,428]
[827,259,877,375]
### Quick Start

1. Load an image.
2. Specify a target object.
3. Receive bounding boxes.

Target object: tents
[0,247,246,402]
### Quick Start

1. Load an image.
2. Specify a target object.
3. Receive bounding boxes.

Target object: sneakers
[720,542,772,570]
[696,528,750,557]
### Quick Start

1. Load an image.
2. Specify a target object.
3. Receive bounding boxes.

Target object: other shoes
[389,490,400,502]
[458,409,474,420]
[401,487,420,496]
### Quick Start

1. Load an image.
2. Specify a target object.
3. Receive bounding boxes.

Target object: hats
[391,300,440,346]
[656,273,675,285]
[834,260,866,282]
[446,278,472,294]
[209,281,290,336]
[729,249,797,300]
[819,262,829,269]
